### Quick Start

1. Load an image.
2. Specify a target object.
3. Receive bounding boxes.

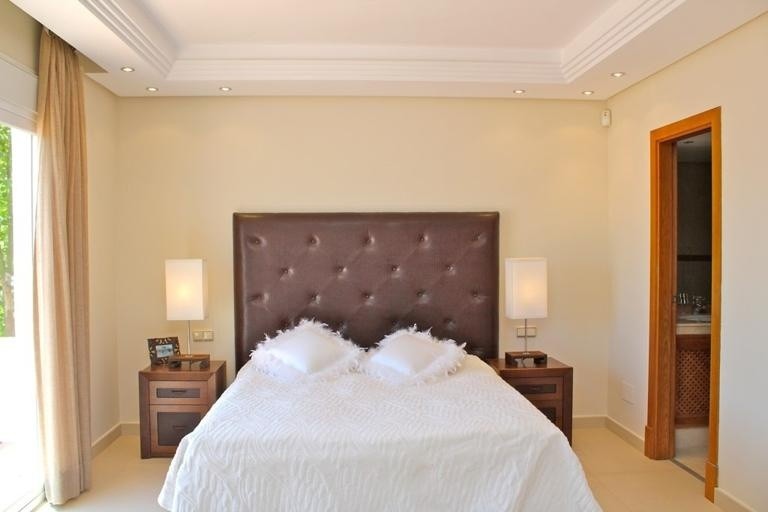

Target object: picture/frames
[146,336,180,370]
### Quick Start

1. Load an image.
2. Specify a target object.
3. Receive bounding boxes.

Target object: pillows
[249,315,467,386]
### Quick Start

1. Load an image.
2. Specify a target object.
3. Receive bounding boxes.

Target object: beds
[175,352,602,512]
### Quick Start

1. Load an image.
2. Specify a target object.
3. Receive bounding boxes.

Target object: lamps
[504,257,549,364]
[163,257,211,369]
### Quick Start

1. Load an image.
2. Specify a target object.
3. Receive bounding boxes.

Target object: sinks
[680,315,710,334]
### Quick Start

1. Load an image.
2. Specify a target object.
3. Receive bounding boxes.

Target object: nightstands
[138,360,227,460]
[482,357,573,450]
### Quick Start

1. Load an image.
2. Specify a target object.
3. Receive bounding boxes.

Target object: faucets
[692,295,707,314]
